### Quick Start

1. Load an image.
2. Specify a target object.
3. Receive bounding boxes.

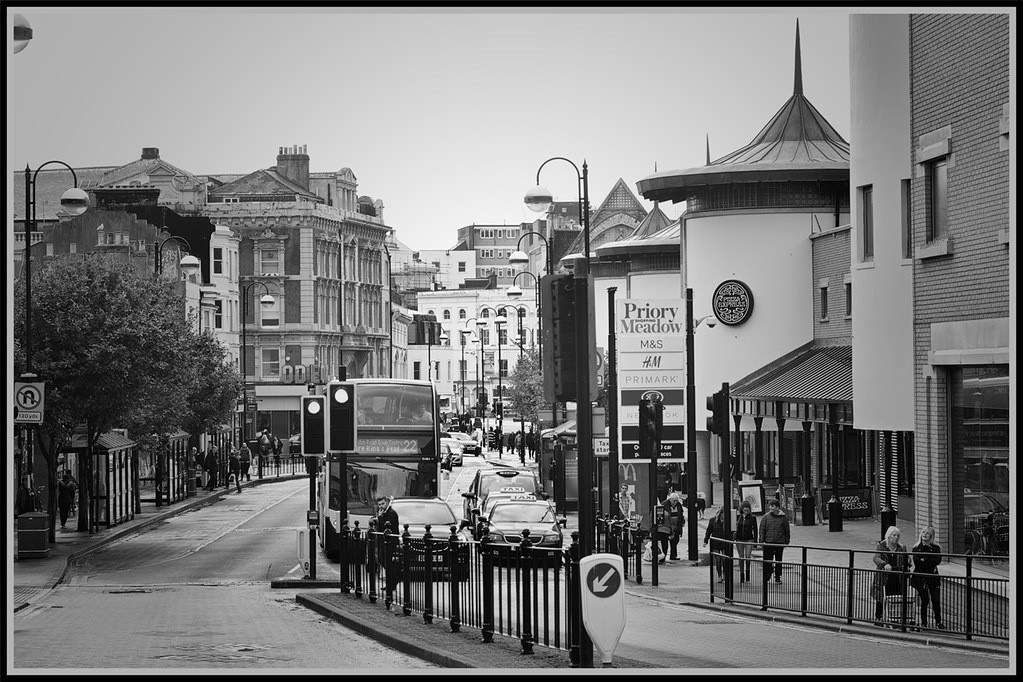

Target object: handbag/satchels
[643,541,665,561]
[733,532,736,540]
[247,465,254,475]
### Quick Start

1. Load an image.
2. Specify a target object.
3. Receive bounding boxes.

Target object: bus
[315,376,442,565]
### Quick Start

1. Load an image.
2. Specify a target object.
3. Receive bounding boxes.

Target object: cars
[442,431,482,457]
[384,495,470,585]
[963,486,1007,527]
[439,437,468,466]
[461,466,568,569]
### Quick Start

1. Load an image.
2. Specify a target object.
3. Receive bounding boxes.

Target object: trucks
[433,380,455,414]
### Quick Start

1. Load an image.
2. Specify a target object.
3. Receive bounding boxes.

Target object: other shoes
[718,577,722,582]
[937,623,945,629]
[775,579,782,584]
[671,557,680,560]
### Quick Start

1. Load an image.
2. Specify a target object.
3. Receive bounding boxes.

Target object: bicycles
[965,507,1009,569]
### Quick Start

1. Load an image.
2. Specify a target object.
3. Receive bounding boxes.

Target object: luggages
[882,566,916,631]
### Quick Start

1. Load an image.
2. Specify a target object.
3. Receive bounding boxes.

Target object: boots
[740,572,744,582]
[746,570,750,581]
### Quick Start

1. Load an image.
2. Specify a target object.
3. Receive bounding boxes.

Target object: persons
[910,526,947,630]
[873,526,908,626]
[191,428,284,492]
[440,410,536,463]
[619,484,632,523]
[650,493,685,565]
[16,478,28,515]
[58,469,106,530]
[759,499,790,584]
[359,394,375,412]
[414,402,432,424]
[533,483,546,500]
[736,501,758,583]
[376,496,401,592]
[703,505,725,583]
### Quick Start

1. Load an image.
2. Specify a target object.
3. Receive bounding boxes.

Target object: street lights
[241,281,276,442]
[685,287,717,561]
[462,303,539,426]
[506,270,542,376]
[508,231,557,429]
[154,236,200,276]
[428,324,448,382]
[24,158,90,472]
[522,154,594,560]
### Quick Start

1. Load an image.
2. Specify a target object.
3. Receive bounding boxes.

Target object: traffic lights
[643,393,664,459]
[705,389,724,439]
[324,381,358,454]
[300,395,327,458]
